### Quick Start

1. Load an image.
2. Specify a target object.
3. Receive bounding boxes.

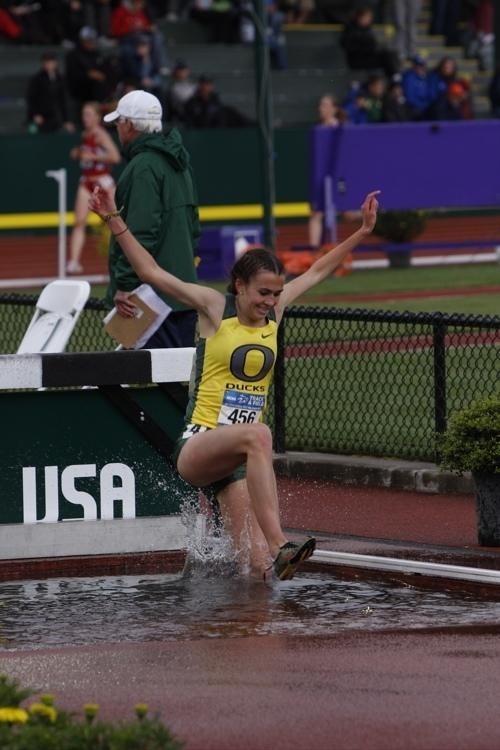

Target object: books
[106,293,158,350]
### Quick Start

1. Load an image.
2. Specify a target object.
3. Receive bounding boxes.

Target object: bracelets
[104,206,127,223]
[113,228,130,236]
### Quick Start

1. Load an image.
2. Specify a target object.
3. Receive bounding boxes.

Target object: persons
[1,1,499,134]
[308,93,347,246]
[65,101,122,275]
[86,177,384,588]
[103,89,201,350]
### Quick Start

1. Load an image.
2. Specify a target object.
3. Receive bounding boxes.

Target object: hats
[101,89,163,123]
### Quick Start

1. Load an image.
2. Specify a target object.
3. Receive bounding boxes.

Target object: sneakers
[272,539,317,581]
